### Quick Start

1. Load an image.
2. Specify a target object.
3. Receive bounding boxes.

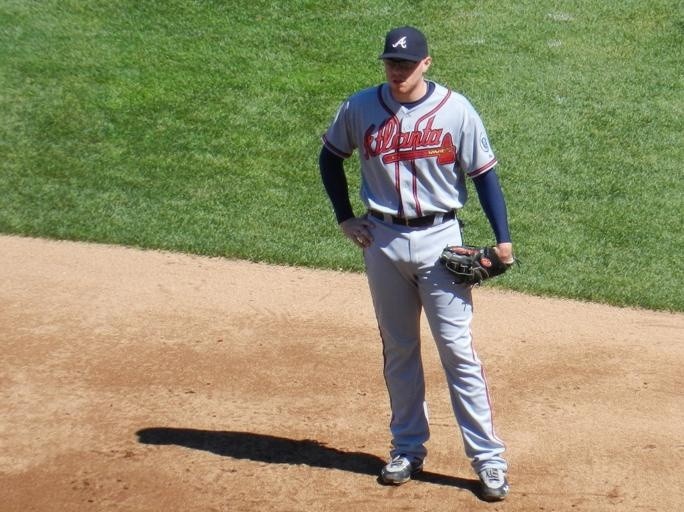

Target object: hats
[376,25,429,64]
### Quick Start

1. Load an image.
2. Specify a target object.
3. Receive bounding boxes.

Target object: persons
[319,27,517,501]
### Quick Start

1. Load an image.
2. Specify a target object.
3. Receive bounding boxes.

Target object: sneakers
[380,453,424,485]
[477,468,510,501]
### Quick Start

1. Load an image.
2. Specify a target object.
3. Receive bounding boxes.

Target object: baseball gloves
[441,245,515,285]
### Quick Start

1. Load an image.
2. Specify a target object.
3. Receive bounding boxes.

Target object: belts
[366,208,456,228]
